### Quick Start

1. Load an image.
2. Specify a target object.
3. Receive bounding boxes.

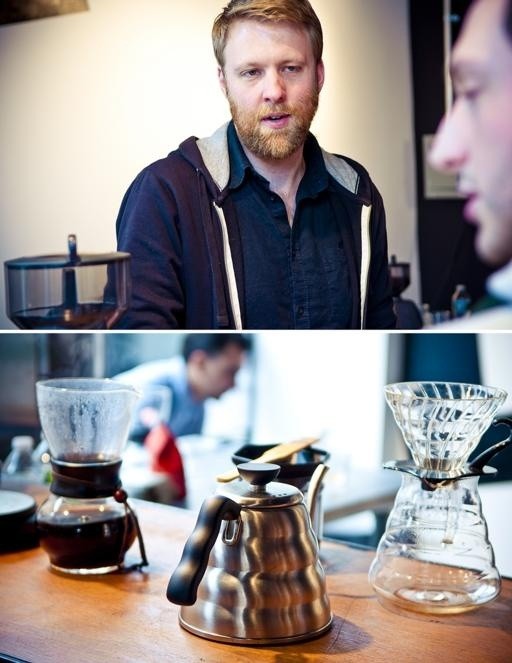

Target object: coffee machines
[4,235,130,335]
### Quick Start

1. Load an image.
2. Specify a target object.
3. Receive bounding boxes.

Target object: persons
[104,0,400,329]
[108,334,251,442]
[425,0,512,312]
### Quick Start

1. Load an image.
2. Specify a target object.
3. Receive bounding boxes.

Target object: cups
[32,378,138,575]
[368,380,512,615]
[230,443,330,569]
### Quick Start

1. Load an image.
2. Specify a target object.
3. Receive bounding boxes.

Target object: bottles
[452,285,472,327]
[1,432,46,486]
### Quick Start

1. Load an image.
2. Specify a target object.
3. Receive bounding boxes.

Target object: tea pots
[168,462,336,646]
